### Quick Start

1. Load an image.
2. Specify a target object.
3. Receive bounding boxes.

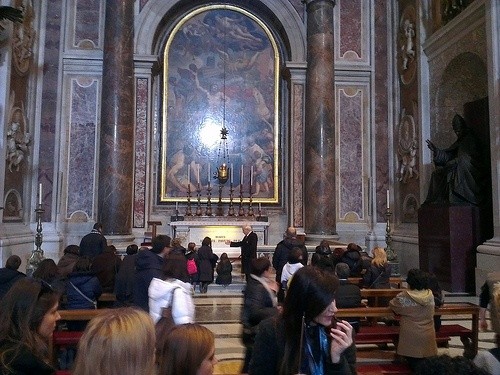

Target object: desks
[170,220,271,246]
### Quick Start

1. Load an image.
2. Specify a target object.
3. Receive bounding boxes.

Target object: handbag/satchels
[90,300,98,309]
[155,287,181,349]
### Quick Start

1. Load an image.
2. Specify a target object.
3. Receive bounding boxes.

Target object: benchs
[53,293,116,375]
[334,277,479,375]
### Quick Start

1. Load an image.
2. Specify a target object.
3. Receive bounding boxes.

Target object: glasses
[36,280,56,301]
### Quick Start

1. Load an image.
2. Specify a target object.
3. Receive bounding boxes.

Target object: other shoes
[203,285,207,293]
[199,282,203,293]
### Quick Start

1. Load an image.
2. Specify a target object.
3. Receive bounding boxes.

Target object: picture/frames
[157,4,282,207]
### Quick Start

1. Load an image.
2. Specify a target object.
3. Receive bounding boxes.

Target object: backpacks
[187,259,197,274]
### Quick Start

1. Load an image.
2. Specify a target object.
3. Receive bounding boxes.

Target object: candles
[231,163,233,182]
[207,163,211,182]
[176,202,178,210]
[250,165,253,185]
[39,183,42,204]
[240,164,243,184]
[198,163,199,183]
[386,190,390,208]
[259,202,261,210]
[188,163,190,183]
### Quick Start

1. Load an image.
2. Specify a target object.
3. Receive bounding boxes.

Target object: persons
[9,123,31,174]
[388,267,438,375]
[223,225,258,275]
[401,18,415,70]
[14,2,34,63]
[423,113,480,206]
[238,265,357,375]
[0,223,500,375]
[398,145,421,184]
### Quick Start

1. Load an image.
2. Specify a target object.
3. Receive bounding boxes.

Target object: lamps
[213,0,230,182]
